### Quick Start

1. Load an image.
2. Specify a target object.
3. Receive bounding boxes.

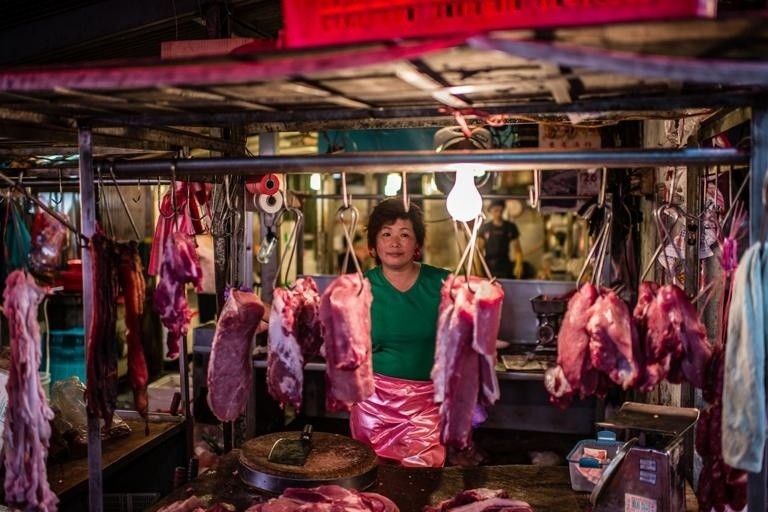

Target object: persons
[348,196,464,466]
[475,196,523,280]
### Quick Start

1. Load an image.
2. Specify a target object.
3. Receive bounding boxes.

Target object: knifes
[268,423,316,466]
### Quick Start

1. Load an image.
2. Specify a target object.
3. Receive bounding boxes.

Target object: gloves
[513,252,523,279]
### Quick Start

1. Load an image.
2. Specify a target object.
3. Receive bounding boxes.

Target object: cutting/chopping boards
[236,431,381,493]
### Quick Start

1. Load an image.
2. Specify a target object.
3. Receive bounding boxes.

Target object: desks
[3,418,181,512]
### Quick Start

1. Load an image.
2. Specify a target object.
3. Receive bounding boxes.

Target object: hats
[487,199,505,212]
